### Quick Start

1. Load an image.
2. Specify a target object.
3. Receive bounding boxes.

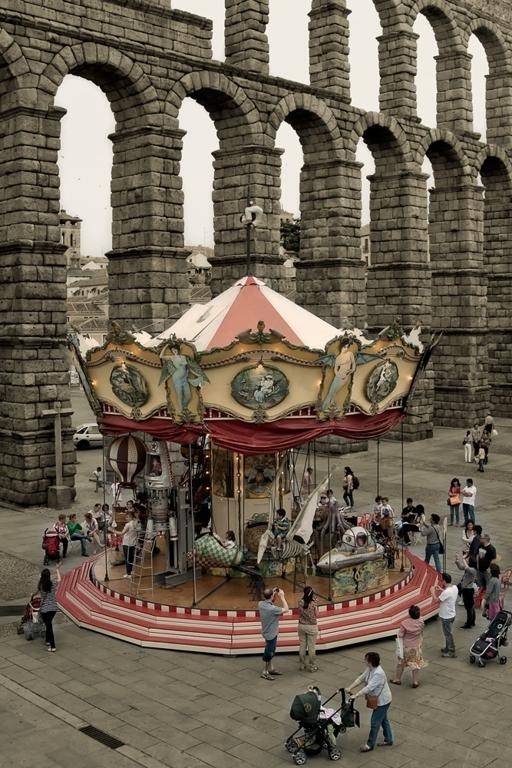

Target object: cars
[73,422,104,451]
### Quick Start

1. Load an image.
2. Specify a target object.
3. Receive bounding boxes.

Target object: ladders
[129,530,158,596]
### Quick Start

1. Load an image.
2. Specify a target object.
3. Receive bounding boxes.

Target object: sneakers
[440,647,454,657]
[460,621,475,628]
[412,680,419,688]
[300,663,306,670]
[82,544,105,556]
[390,679,401,685]
[448,523,458,527]
[261,669,283,680]
[309,664,318,672]
[124,574,132,578]
[46,641,56,652]
[360,744,372,752]
[377,740,388,746]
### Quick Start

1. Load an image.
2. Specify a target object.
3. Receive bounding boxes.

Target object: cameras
[275,588,279,592]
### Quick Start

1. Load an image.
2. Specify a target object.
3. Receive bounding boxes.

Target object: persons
[321,340,356,409]
[342,466,355,508]
[462,411,495,472]
[460,478,477,526]
[346,651,394,753]
[447,478,463,527]
[51,489,504,629]
[386,603,426,689]
[302,467,314,491]
[297,585,321,671]
[36,558,66,652]
[429,572,459,659]
[159,342,201,412]
[93,467,105,492]
[258,376,268,391]
[257,586,290,680]
[252,374,274,405]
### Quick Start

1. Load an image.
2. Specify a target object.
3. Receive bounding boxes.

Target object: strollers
[469,610,511,667]
[42,527,62,565]
[284,685,355,765]
[16,591,47,641]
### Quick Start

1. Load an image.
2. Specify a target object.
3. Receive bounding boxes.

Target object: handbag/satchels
[457,583,462,597]
[463,440,467,445]
[439,543,444,553]
[395,638,404,660]
[366,695,378,708]
[450,496,460,505]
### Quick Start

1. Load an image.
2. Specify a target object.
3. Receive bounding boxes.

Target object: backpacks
[353,477,359,489]
